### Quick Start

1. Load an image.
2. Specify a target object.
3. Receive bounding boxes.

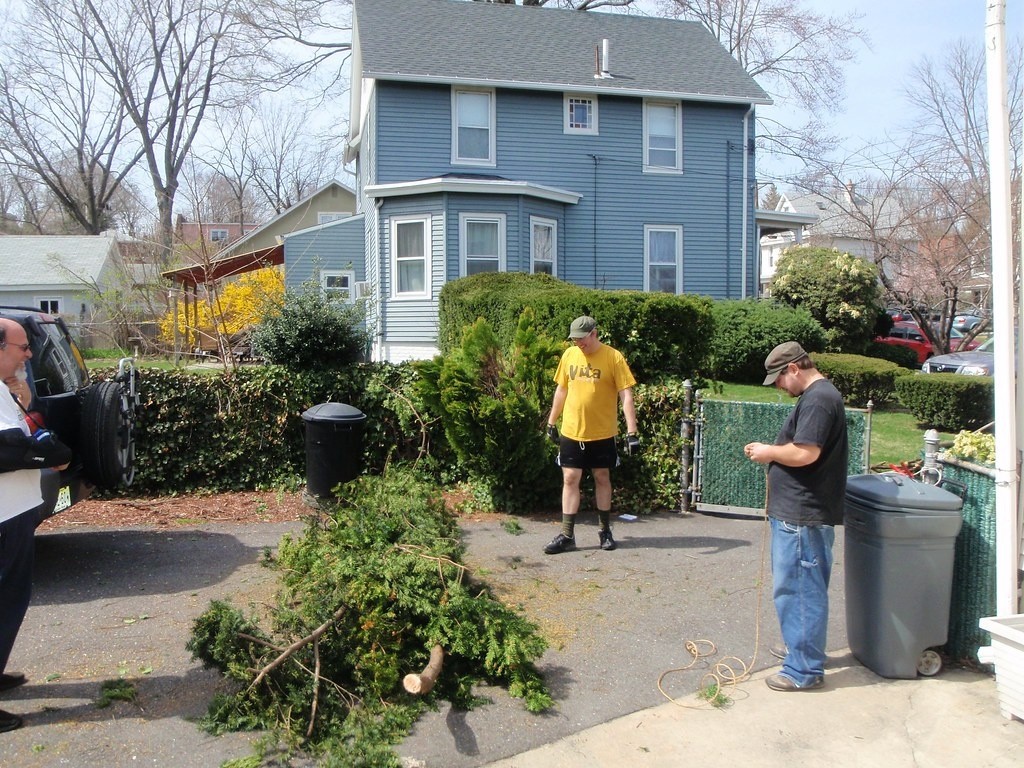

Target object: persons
[542,317,642,555]
[0,318,71,733]
[744,342,849,693]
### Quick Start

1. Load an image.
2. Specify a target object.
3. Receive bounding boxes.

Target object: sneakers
[598,528,615,549]
[765,673,826,691]
[543,531,576,553]
[770,646,830,663]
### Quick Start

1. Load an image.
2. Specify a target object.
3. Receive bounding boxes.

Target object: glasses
[4,341,33,351]
[572,328,595,344]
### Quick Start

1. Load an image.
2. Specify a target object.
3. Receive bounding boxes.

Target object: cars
[922,336,994,376]
[875,321,981,369]
[884,306,992,331]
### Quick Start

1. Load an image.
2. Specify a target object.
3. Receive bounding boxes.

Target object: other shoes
[0,709,21,733]
[0,671,25,691]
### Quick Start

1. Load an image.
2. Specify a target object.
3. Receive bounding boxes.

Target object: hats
[567,316,596,339]
[762,341,807,385]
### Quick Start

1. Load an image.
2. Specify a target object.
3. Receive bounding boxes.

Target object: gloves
[626,432,640,455]
[547,424,560,445]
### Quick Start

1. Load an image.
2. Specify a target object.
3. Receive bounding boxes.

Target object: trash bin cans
[298,400,367,498]
[838,473,968,678]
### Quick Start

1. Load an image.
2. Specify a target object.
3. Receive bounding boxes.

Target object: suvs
[0,305,137,519]
